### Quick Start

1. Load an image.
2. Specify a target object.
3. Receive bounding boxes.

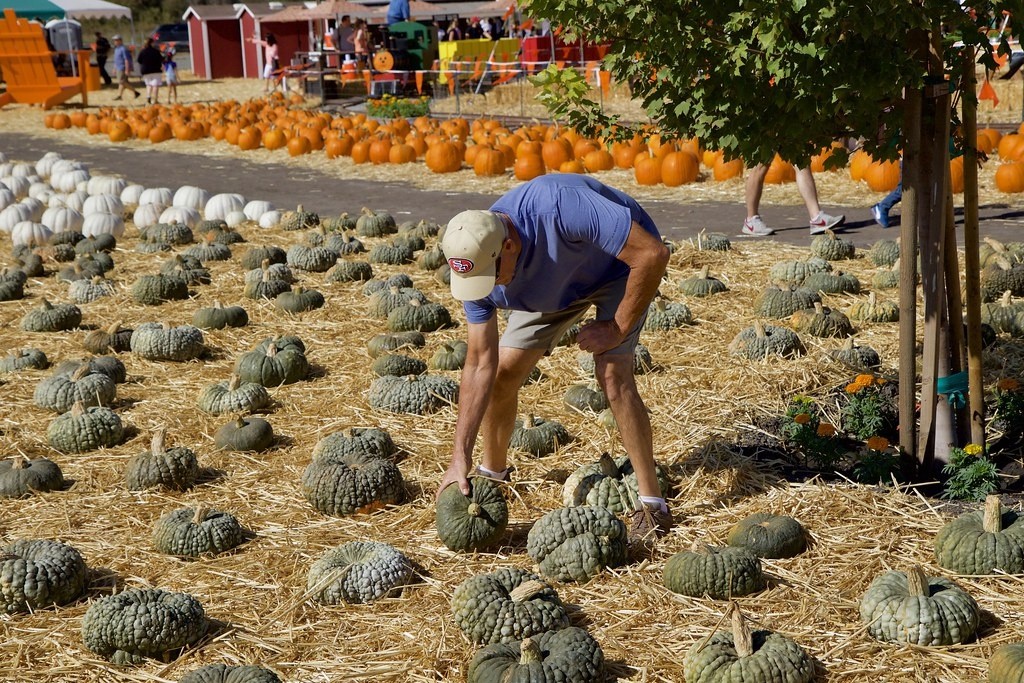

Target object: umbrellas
[258,5,336,21]
[477,0,532,10]
[297,0,373,29]
[372,0,446,12]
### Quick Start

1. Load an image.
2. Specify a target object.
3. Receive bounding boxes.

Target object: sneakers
[468,464,516,485]
[740,214,776,236]
[626,503,674,559]
[870,203,891,229]
[809,210,846,235]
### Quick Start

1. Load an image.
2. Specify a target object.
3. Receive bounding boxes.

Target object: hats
[469,17,481,24]
[166,51,173,57]
[110,34,123,41]
[441,207,508,303]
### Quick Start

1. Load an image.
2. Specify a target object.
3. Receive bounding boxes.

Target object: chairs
[0,8,93,110]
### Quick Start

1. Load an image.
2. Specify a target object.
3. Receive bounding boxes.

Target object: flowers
[365,94,430,118]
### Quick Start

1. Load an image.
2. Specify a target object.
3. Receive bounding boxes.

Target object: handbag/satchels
[269,60,283,80]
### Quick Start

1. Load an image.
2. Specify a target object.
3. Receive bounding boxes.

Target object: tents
[0,0,141,77]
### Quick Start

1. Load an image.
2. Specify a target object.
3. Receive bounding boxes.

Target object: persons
[161,52,181,104]
[739,131,846,238]
[138,38,165,104]
[387,0,415,25]
[111,34,140,100]
[244,33,281,91]
[94,31,112,86]
[436,173,674,545]
[432,16,537,60]
[869,153,903,229]
[48,44,59,66]
[322,15,406,71]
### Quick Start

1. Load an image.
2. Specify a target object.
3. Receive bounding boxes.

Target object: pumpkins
[43,88,1023,197]
[0,151,1024,683]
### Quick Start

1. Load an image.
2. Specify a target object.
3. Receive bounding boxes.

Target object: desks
[295,50,376,107]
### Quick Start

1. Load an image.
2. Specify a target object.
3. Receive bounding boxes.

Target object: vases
[367,116,416,125]
